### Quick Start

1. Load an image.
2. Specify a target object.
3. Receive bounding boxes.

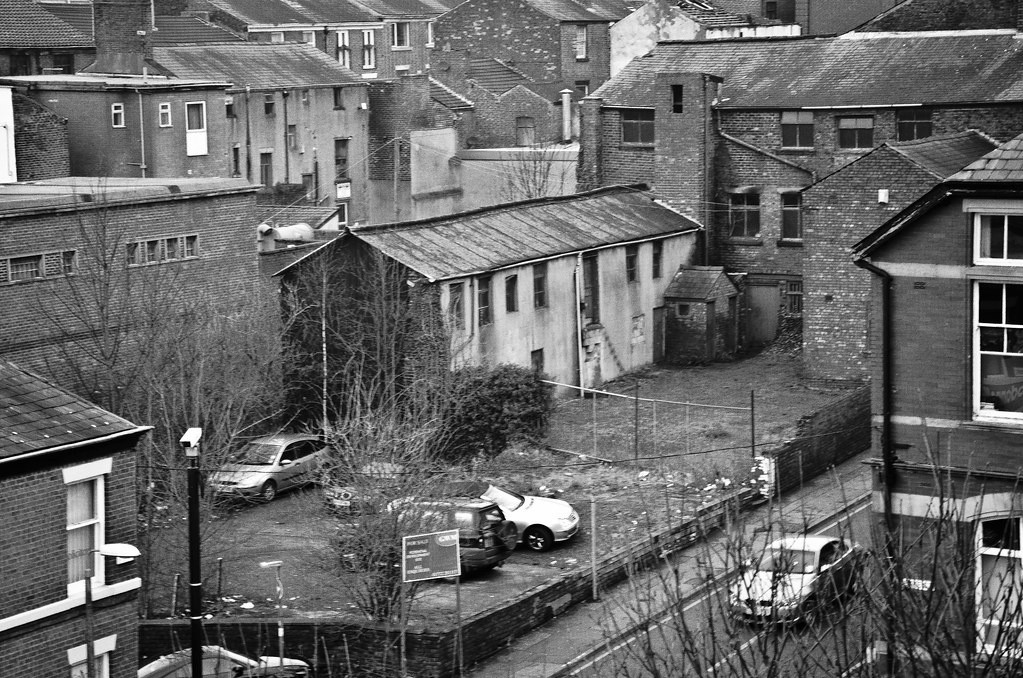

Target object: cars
[136,645,311,678]
[322,462,413,518]
[723,534,865,632]
[386,481,581,554]
[207,434,333,504]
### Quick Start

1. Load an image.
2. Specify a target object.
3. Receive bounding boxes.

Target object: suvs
[325,496,518,586]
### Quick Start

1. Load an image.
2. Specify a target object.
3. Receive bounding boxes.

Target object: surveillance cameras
[178,426,203,448]
